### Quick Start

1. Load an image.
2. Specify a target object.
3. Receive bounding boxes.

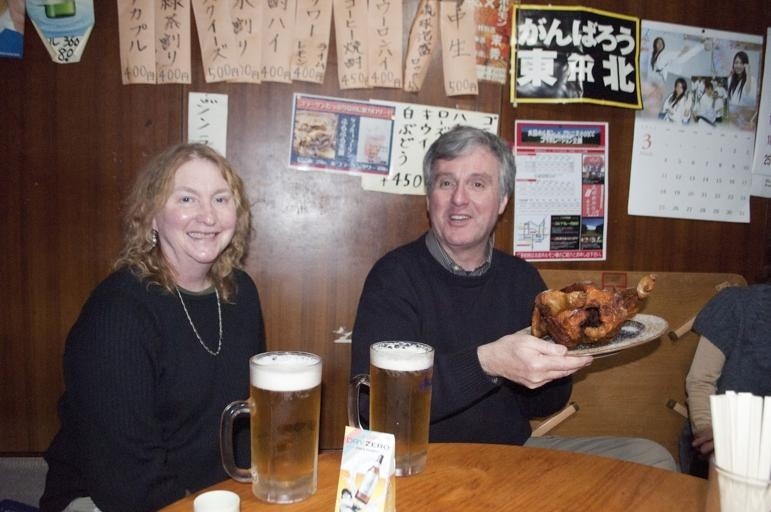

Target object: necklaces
[170,276,224,356]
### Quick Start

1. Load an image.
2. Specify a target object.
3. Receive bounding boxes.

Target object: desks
[525,267,753,476]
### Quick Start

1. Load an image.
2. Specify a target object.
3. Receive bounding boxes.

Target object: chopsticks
[710,390,771,483]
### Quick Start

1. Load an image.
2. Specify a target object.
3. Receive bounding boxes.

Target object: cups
[348,340,435,480]
[194,488,240,511]
[219,352,322,503]
[707,456,771,512]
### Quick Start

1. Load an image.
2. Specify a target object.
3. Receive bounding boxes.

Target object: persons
[349,126,677,473]
[678,284,771,480]
[40,143,266,512]
[646,37,757,128]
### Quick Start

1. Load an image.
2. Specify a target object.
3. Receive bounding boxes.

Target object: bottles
[354,455,385,504]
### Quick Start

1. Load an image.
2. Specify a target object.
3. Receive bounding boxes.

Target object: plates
[512,312,670,360]
